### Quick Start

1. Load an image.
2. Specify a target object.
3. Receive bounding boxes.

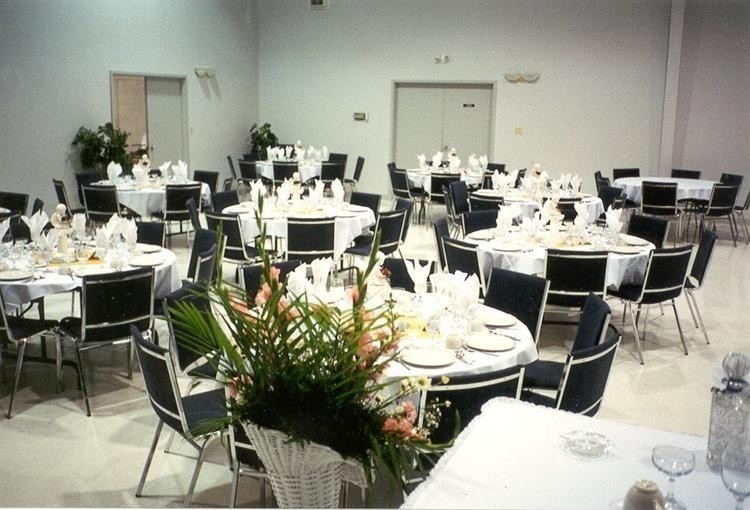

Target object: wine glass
[652,445,696,510]
[720,438,750,510]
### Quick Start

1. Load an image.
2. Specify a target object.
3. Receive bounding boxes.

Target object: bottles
[706,352,750,474]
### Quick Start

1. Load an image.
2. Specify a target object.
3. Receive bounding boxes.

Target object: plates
[469,219,650,254]
[1,232,163,280]
[231,199,368,220]
[393,294,519,367]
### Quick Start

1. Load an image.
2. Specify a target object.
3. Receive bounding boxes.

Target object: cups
[328,276,359,303]
[621,479,665,510]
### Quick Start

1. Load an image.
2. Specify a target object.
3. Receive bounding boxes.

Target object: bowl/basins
[566,431,608,457]
